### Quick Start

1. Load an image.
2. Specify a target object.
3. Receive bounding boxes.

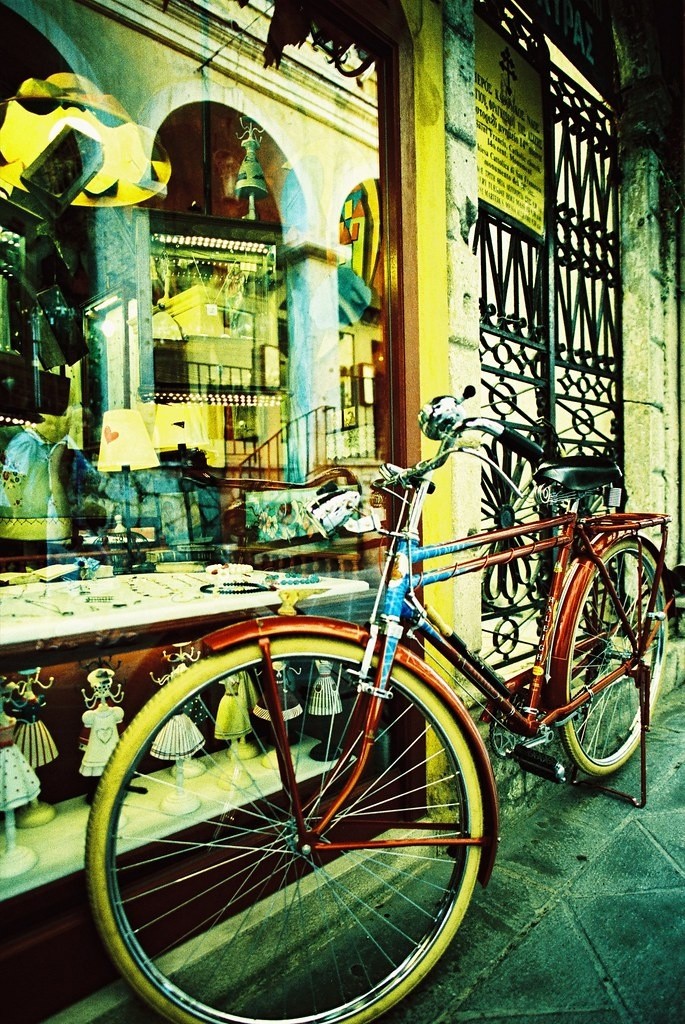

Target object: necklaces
[0,563,320,617]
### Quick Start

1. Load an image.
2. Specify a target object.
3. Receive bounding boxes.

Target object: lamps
[96,408,160,576]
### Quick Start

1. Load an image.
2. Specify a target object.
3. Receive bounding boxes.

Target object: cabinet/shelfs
[0,564,403,1024]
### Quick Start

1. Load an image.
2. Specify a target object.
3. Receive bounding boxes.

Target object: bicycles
[83,383,677,1023]
